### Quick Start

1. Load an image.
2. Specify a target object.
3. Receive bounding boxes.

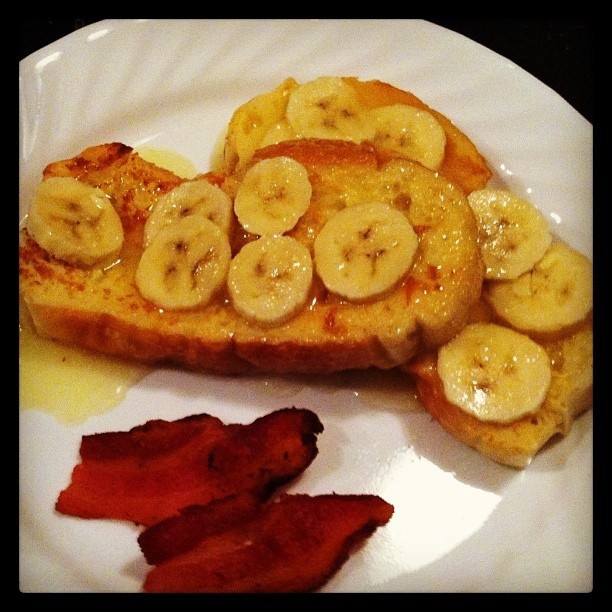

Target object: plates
[21,20,594,591]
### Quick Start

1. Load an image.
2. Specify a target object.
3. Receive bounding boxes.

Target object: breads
[223,77,593,470]
[19,144,483,373]
